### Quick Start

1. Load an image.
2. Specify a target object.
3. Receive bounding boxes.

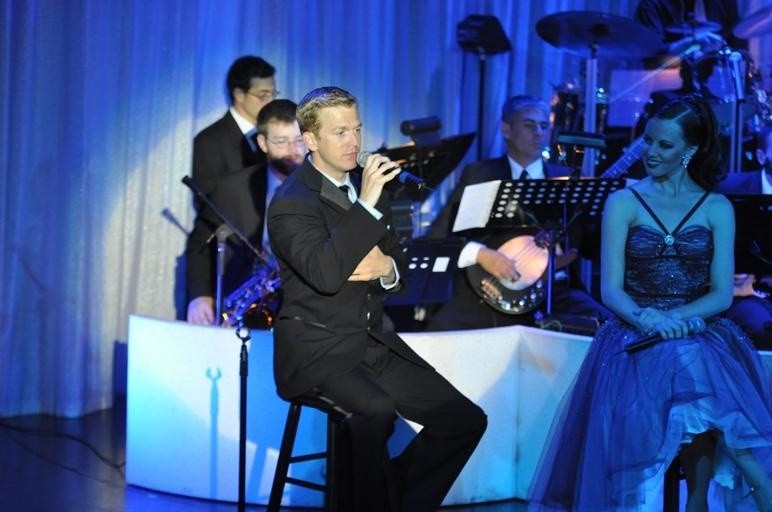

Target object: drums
[681,50,746,103]
[553,84,610,166]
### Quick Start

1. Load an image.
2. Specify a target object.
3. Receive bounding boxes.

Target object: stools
[265,386,397,512]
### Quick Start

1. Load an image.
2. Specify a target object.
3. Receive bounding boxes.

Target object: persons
[598,94,772,512]
[185,99,309,325]
[266,84,491,512]
[192,53,280,208]
[424,94,616,338]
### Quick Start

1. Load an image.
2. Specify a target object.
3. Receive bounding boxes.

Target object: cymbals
[535,11,661,59]
[731,3,771,39]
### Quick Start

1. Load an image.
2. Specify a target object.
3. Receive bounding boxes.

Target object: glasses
[264,137,304,148]
[244,90,280,101]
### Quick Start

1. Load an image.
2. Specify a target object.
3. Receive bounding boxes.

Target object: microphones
[729,51,746,104]
[356,151,426,192]
[624,316,705,356]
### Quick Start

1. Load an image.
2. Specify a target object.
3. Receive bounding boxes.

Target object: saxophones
[213,159,303,328]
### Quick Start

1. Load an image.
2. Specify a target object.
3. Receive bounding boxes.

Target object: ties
[338,186,348,197]
[520,170,527,180]
